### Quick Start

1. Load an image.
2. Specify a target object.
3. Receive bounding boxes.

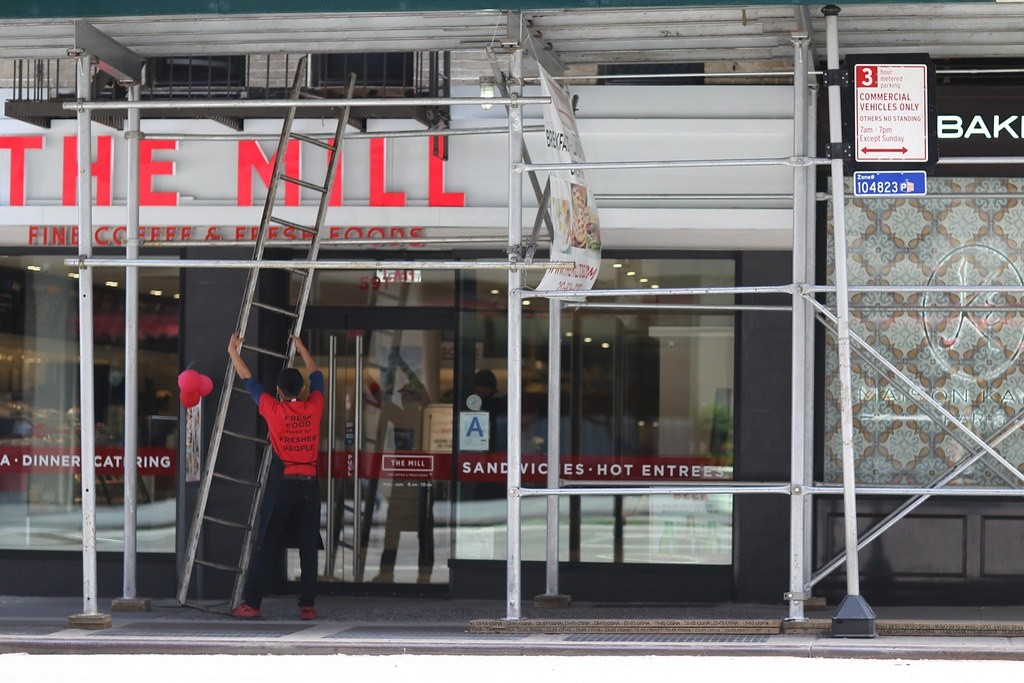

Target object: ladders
[321,267,416,584]
[173,55,359,623]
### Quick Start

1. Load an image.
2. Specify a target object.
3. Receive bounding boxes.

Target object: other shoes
[300,604,320,620]
[232,602,262,617]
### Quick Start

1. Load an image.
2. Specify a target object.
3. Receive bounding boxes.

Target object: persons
[359,346,436,587]
[228,325,325,620]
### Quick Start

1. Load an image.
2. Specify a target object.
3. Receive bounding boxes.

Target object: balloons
[177,369,213,408]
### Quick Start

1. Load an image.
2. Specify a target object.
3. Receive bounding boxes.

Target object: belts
[279,473,318,481]
